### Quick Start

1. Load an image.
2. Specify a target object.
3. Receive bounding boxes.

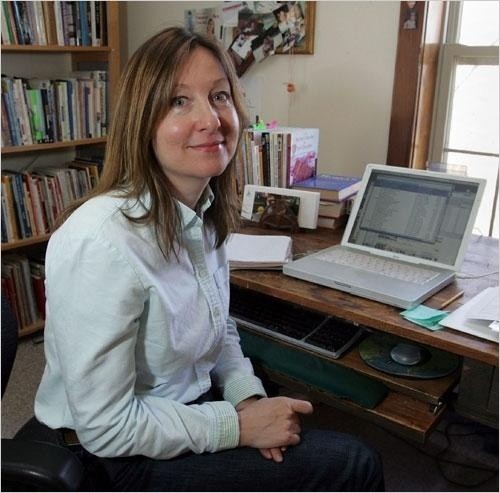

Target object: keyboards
[229,283,365,359]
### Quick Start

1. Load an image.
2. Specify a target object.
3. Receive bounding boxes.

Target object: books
[0,0,110,333]
[228,124,364,231]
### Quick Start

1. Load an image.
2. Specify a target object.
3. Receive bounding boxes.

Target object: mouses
[389,339,422,366]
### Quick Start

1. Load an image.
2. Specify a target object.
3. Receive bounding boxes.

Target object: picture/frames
[232,1,317,55]
[241,184,320,229]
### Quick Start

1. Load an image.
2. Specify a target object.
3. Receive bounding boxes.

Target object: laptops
[282,162,487,311]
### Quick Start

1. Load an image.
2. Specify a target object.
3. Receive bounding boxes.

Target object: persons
[185,0,305,61]
[31,24,382,489]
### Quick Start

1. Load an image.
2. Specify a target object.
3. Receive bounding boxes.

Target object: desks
[229,227,499,444]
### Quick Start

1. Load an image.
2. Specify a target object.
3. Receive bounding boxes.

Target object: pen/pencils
[437,290,465,310]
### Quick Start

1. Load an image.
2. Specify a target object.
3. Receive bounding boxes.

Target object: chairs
[1,299,113,492]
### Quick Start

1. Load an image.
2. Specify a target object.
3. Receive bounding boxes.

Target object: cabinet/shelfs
[1,1,129,346]
